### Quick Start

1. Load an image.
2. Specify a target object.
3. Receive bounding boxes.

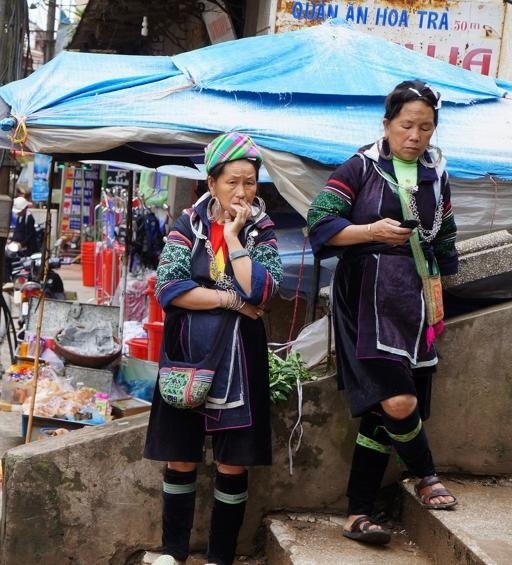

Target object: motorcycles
[3,239,65,328]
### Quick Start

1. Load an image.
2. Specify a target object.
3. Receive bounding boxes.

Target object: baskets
[55,325,122,367]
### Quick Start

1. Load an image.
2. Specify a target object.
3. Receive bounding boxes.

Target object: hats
[205,132,263,174]
[12,197,32,214]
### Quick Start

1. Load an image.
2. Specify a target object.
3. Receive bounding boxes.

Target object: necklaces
[185,208,258,291]
[372,159,444,243]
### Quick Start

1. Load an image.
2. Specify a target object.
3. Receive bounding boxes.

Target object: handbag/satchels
[158,367,215,409]
[424,272,444,325]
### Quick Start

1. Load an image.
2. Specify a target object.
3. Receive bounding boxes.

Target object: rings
[256,310,261,316]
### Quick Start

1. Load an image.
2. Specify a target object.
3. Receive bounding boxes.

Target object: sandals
[414,474,458,509]
[343,515,390,544]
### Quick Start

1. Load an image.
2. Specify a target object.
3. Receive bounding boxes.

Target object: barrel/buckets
[124,275,167,364]
[81,241,96,287]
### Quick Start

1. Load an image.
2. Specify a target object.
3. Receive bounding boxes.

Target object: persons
[142,131,285,565]
[10,196,40,257]
[9,185,31,223]
[304,78,462,548]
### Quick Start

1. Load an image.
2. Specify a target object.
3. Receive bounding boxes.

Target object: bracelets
[213,287,247,312]
[368,223,372,242]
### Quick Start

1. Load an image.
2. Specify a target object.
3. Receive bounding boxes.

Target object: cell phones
[398,219,419,230]
